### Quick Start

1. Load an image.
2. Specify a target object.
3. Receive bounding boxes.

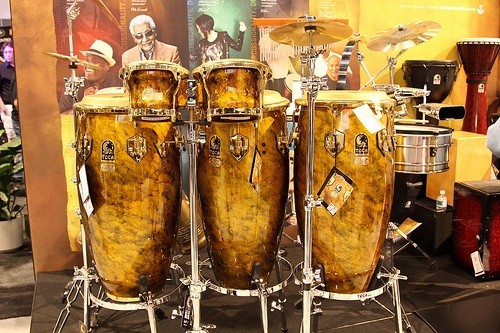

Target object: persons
[314,49,342,79]
[0,42,23,165]
[195,13,247,66]
[320,55,346,90]
[59,39,123,112]
[485,117,500,180]
[121,15,182,68]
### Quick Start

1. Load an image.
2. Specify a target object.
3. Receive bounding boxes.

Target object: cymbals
[43,52,104,71]
[366,21,442,52]
[266,20,353,46]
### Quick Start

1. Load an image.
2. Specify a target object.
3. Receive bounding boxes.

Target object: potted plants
[0,144,26,253]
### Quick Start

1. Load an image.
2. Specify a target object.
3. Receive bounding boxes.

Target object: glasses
[131,29,154,40]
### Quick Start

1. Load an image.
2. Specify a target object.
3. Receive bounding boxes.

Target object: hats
[78,40,117,67]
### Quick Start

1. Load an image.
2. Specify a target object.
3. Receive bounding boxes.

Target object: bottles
[435,190,447,213]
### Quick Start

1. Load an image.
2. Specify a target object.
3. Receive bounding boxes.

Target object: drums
[401,59,459,123]
[72,94,182,303]
[196,88,291,292]
[295,88,394,296]
[394,123,454,174]
[119,60,189,124]
[456,37,500,135]
[190,58,272,123]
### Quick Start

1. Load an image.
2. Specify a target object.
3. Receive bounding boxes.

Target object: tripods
[53,271,165,333]
